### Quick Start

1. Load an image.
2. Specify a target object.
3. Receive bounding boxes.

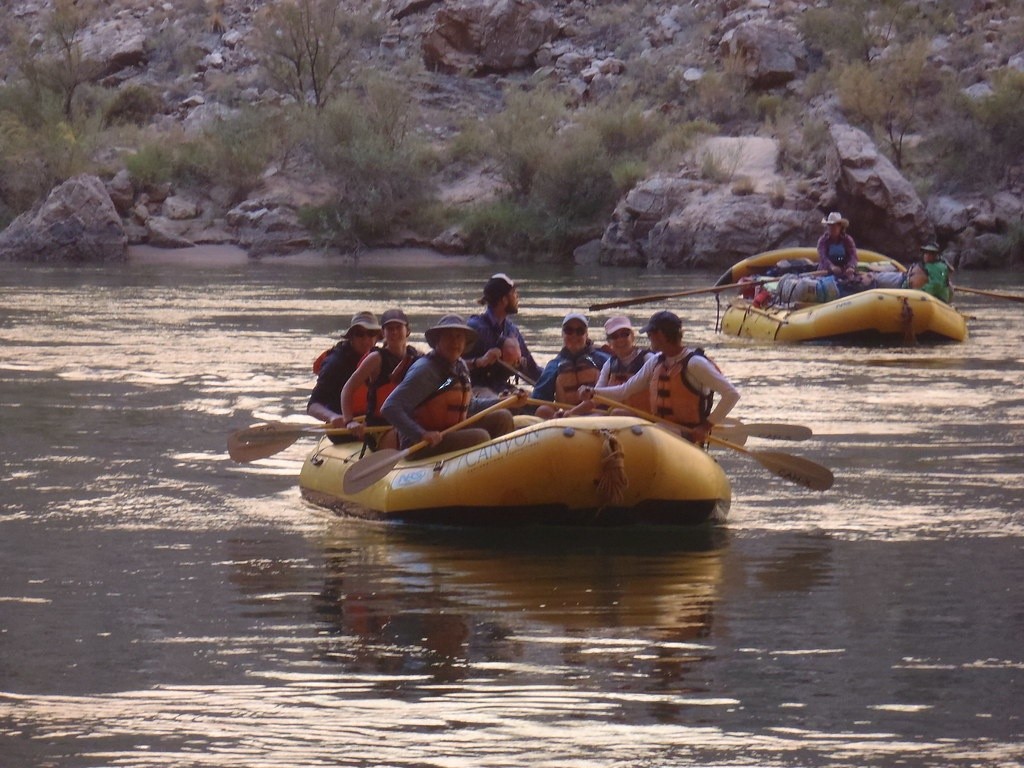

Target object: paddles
[588,269,833,312]
[225,414,394,465]
[526,397,813,441]
[342,387,525,495]
[585,386,835,492]
[954,287,1023,302]
[495,357,748,448]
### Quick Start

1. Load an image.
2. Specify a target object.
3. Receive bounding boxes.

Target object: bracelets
[345,419,353,427]
[471,358,478,369]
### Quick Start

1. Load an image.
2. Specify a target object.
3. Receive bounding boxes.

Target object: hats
[822,213,849,228]
[483,272,521,301]
[562,314,588,327]
[341,311,383,339]
[639,311,681,333]
[605,315,632,336]
[381,309,408,329]
[424,314,479,353]
[921,242,939,252]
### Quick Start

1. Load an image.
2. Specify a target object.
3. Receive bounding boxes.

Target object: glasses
[352,330,378,337]
[607,332,630,340]
[647,331,657,337]
[563,325,586,336]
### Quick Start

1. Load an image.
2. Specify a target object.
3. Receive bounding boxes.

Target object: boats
[713,245,969,347]
[297,413,732,526]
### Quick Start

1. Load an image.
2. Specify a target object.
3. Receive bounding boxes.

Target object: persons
[577,311,741,448]
[815,211,857,278]
[307,310,384,445]
[523,313,611,421]
[908,242,953,304]
[340,310,426,452]
[553,315,656,419]
[380,313,527,463]
[459,273,545,400]
